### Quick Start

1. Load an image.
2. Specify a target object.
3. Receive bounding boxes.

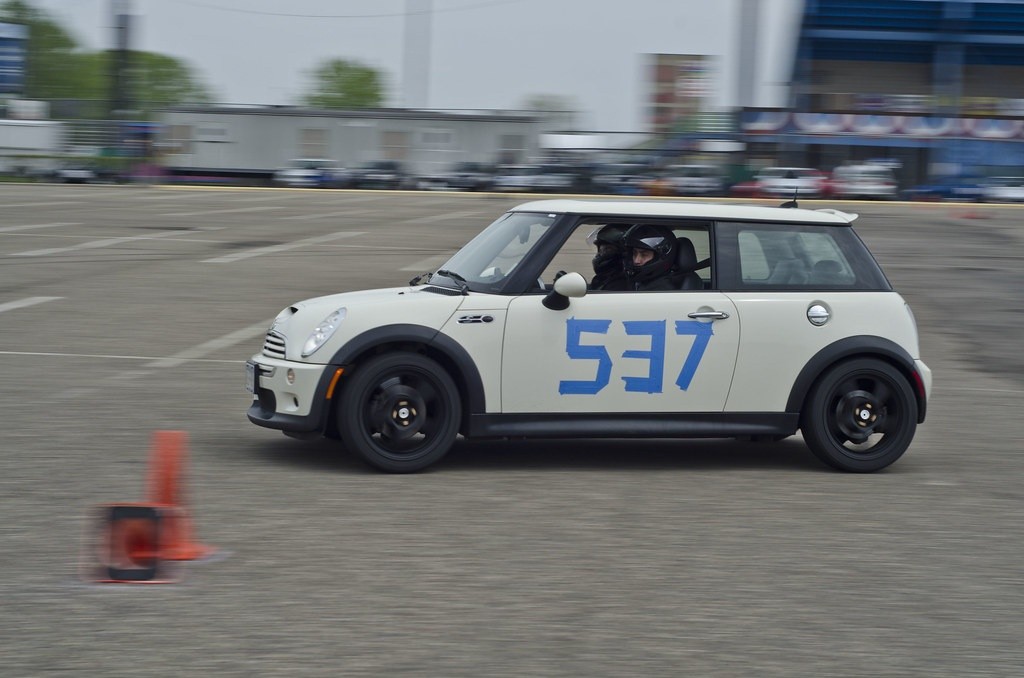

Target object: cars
[273,159,899,196]
[246,198,932,472]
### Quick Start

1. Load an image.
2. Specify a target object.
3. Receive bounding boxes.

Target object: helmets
[621,225,679,284]
[592,224,634,277]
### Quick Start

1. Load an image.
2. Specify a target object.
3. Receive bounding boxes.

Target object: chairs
[668,237,704,290]
[766,256,844,285]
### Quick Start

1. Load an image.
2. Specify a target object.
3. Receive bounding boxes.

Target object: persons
[619,223,683,291]
[591,222,634,290]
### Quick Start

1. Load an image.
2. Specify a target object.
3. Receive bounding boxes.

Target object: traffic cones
[83,431,216,585]
